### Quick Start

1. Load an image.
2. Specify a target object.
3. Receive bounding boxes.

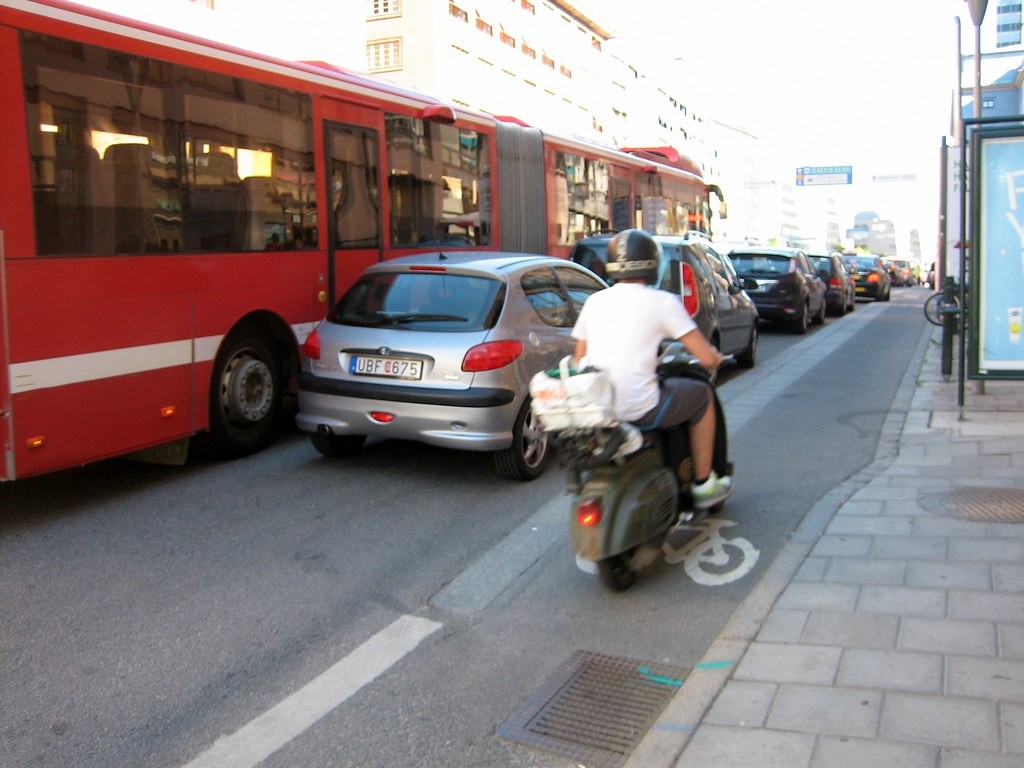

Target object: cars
[727,246,827,334]
[806,249,856,319]
[296,250,611,482]
[845,254,893,303]
[882,260,914,287]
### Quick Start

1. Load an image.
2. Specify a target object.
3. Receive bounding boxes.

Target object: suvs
[567,228,760,388]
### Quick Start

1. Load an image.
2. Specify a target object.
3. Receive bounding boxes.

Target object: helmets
[606,229,659,286]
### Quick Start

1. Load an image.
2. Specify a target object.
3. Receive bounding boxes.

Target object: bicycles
[924,292,967,330]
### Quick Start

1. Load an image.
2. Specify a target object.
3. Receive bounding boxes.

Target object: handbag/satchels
[529,356,619,430]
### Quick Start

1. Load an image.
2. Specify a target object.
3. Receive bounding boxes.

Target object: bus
[1,0,727,484]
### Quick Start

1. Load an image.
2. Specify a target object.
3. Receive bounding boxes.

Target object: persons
[572,229,734,508]
[914,263,921,285]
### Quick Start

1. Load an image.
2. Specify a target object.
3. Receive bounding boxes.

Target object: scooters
[528,341,737,593]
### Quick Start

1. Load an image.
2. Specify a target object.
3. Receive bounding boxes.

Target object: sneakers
[575,552,598,574]
[691,471,735,508]
